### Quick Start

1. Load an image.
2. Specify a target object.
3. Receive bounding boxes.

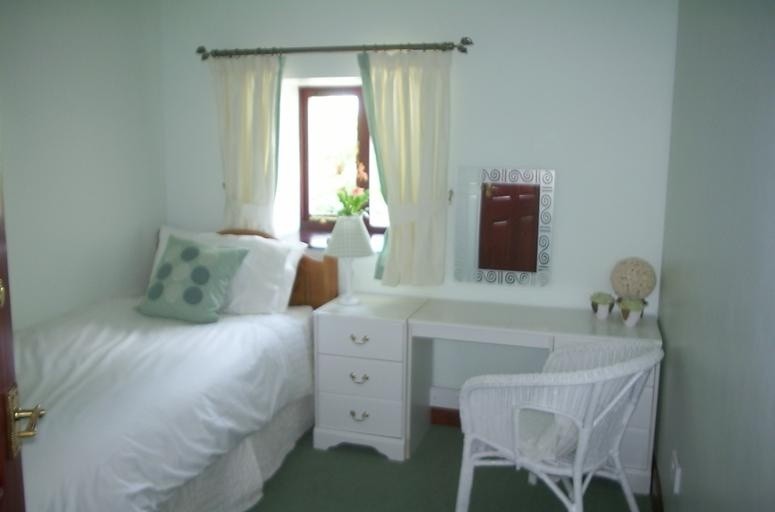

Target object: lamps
[324,216,376,305]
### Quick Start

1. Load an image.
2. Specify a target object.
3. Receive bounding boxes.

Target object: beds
[14,229,337,512]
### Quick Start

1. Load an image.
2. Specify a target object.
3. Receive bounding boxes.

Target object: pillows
[133,227,309,323]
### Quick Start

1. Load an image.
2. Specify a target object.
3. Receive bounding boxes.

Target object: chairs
[454,342,664,512]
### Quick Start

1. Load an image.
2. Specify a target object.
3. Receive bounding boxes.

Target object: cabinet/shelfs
[312,295,664,496]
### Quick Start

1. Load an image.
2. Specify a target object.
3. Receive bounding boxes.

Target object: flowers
[336,161,374,215]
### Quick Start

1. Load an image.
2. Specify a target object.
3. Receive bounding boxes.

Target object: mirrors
[454,168,554,288]
